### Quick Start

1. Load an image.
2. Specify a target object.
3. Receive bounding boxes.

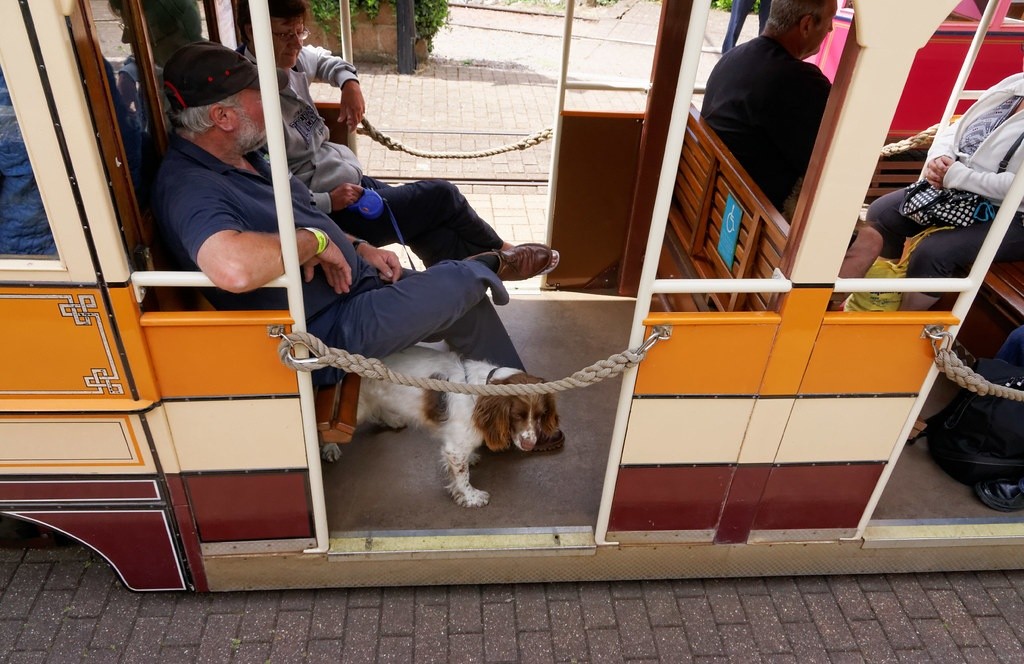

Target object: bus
[1,1,1023,592]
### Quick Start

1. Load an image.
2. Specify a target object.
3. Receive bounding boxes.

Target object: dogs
[320,347,558,507]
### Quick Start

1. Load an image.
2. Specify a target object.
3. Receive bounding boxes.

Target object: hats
[162,42,290,113]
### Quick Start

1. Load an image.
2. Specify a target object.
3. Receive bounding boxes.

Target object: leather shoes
[461,243,553,281]
[975,480,1024,512]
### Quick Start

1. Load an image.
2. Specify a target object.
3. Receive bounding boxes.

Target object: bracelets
[298,227,329,254]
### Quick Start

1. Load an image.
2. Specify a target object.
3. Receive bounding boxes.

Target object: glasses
[273,27,311,42]
[118,21,131,33]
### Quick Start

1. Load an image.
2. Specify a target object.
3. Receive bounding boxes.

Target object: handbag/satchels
[898,178,990,229]
[843,224,955,313]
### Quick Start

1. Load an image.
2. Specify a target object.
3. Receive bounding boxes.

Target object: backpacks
[907,357,1024,485]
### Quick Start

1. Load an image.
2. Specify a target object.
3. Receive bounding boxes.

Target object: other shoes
[509,246,561,274]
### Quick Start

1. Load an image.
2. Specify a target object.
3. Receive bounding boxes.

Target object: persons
[0,0,211,256]
[229,1,559,278]
[867,72,1024,311]
[974,326,1024,513]
[699,0,884,312]
[151,41,565,453]
